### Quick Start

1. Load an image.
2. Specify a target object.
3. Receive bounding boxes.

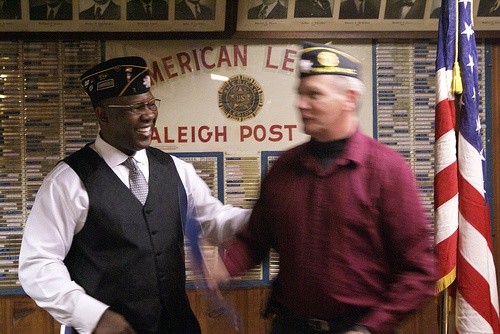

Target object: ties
[121,157,149,206]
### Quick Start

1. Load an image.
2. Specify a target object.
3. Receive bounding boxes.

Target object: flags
[436,0,499,334]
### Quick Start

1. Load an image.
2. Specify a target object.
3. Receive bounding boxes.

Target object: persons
[19,54,254,333]
[202,40,438,334]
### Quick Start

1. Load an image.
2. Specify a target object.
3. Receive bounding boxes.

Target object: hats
[299,47,365,80]
[79,56,152,101]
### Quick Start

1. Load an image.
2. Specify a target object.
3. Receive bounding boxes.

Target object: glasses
[104,98,161,112]
